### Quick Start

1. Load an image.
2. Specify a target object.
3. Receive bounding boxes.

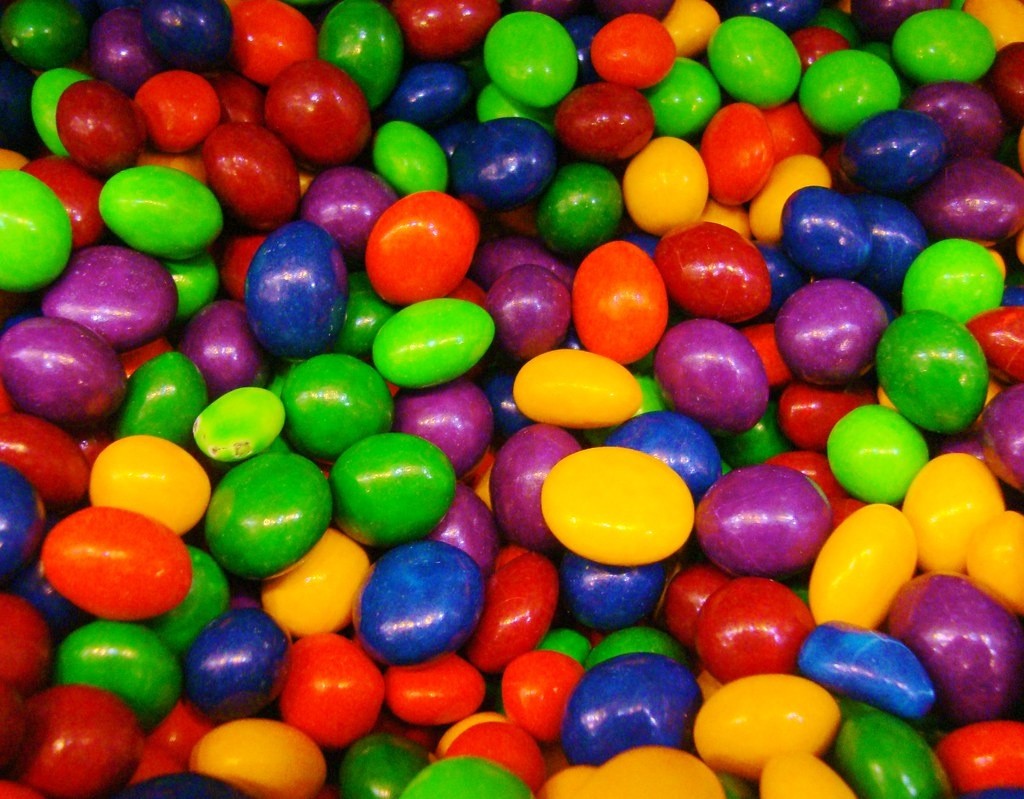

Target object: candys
[0,0,1024,799]
